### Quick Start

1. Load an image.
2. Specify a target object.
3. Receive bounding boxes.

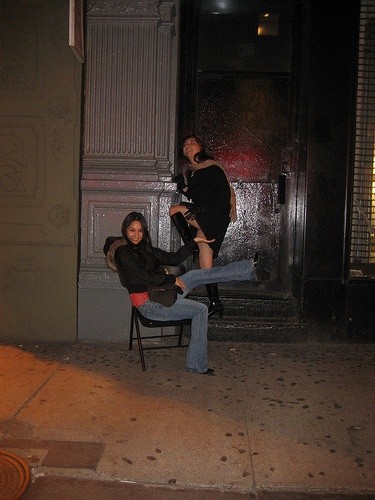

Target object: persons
[114,211,262,376]
[167,136,232,321]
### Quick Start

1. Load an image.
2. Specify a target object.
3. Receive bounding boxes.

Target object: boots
[171,211,201,264]
[205,283,223,321]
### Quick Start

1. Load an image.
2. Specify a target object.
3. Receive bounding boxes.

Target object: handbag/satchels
[147,284,184,308]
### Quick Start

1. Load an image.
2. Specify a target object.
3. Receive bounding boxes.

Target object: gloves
[173,173,188,190]
[184,205,202,221]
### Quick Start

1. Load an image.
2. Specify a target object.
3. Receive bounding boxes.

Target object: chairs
[130,264,193,372]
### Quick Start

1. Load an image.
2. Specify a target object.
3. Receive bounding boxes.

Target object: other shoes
[253,253,260,269]
[203,368,214,374]
